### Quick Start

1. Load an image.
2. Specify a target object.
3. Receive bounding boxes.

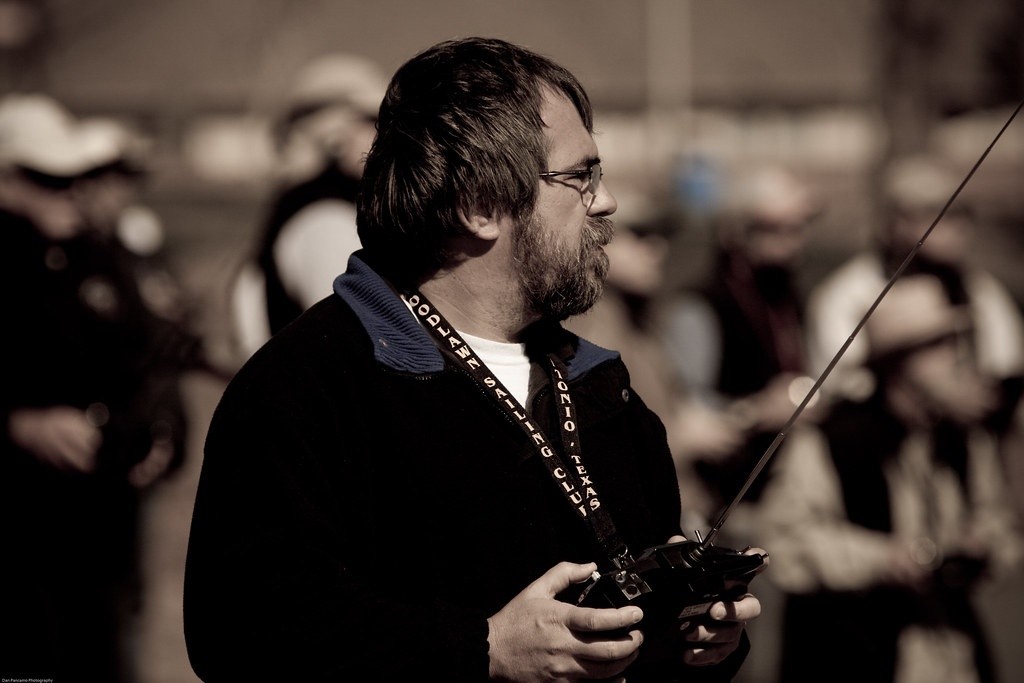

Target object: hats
[734,174,816,232]
[1,91,116,177]
[843,276,984,369]
[889,159,972,210]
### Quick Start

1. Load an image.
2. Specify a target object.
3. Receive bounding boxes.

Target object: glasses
[538,163,604,195]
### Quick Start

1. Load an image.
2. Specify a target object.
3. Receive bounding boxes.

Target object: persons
[228,52,390,369]
[0,85,200,683]
[560,104,1024,683]
[183,37,769,683]
